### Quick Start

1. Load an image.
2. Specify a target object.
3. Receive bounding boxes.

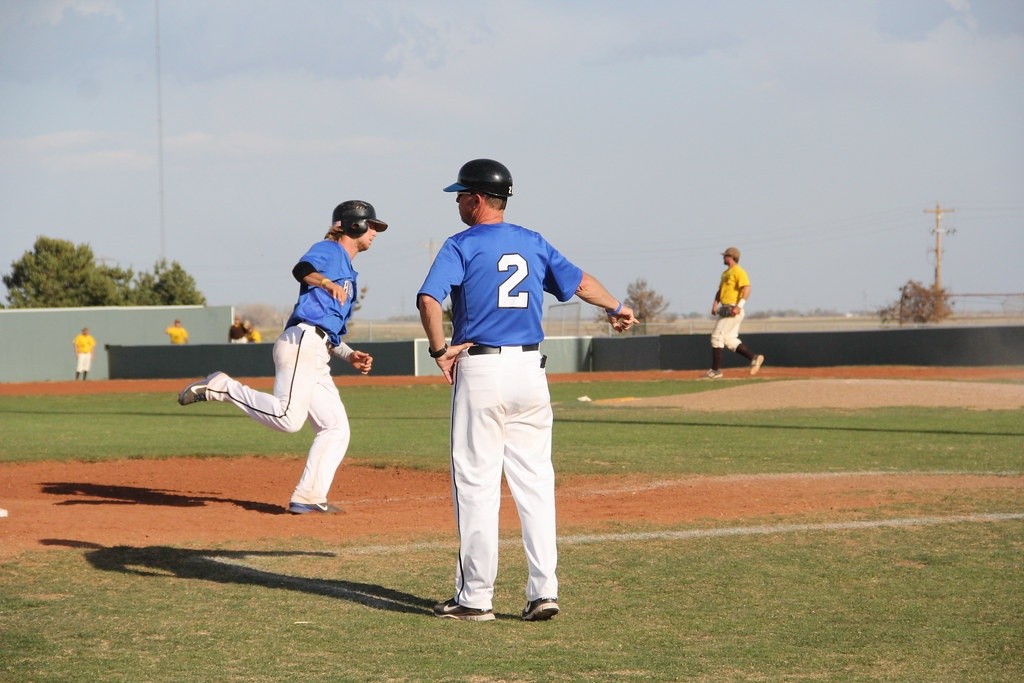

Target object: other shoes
[699,368,723,379]
[749,353,764,375]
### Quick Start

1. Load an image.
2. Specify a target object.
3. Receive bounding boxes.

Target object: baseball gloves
[716,305,736,317]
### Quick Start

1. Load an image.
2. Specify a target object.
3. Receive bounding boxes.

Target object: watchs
[428,343,448,358]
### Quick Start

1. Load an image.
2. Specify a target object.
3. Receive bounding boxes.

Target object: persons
[72,328,96,381]
[228,316,248,344]
[178,200,388,514]
[164,320,190,345]
[243,319,262,343]
[707,247,764,379]
[416,159,640,623]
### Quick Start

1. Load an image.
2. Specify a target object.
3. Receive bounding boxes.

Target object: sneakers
[178,370,223,405]
[288,502,347,514]
[522,598,560,620]
[433,598,496,621]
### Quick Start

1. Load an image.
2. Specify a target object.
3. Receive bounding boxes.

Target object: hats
[720,248,740,262]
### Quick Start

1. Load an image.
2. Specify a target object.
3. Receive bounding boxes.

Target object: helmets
[442,159,514,196]
[331,200,388,240]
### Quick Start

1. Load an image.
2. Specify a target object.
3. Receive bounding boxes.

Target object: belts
[292,322,335,355]
[469,342,538,354]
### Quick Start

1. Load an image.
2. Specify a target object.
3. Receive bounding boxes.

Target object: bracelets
[605,300,622,316]
[321,279,331,288]
